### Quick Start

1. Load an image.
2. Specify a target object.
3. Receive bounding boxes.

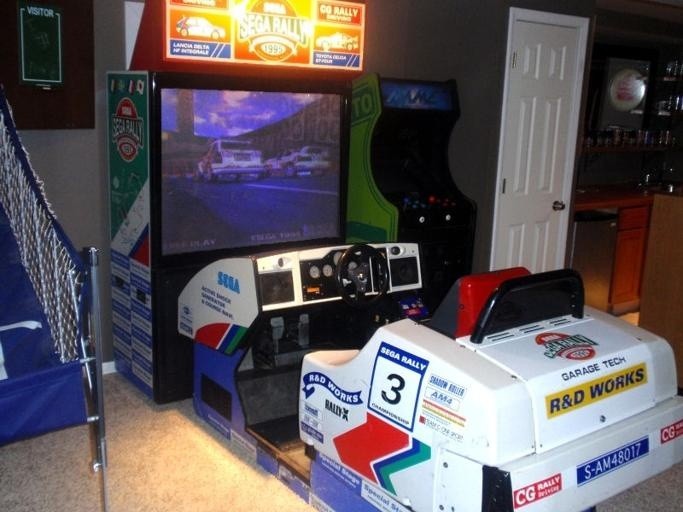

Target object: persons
[177,16,188,28]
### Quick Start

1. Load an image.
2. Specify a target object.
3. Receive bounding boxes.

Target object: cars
[196,140,333,180]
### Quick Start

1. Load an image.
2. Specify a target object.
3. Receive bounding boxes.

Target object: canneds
[614,128,672,147]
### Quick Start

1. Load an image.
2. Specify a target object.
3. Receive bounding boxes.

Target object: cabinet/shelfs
[571,203,654,317]
[575,14,682,187]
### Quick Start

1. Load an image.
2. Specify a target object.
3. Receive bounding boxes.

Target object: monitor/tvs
[372,127,440,187]
[161,88,342,256]
